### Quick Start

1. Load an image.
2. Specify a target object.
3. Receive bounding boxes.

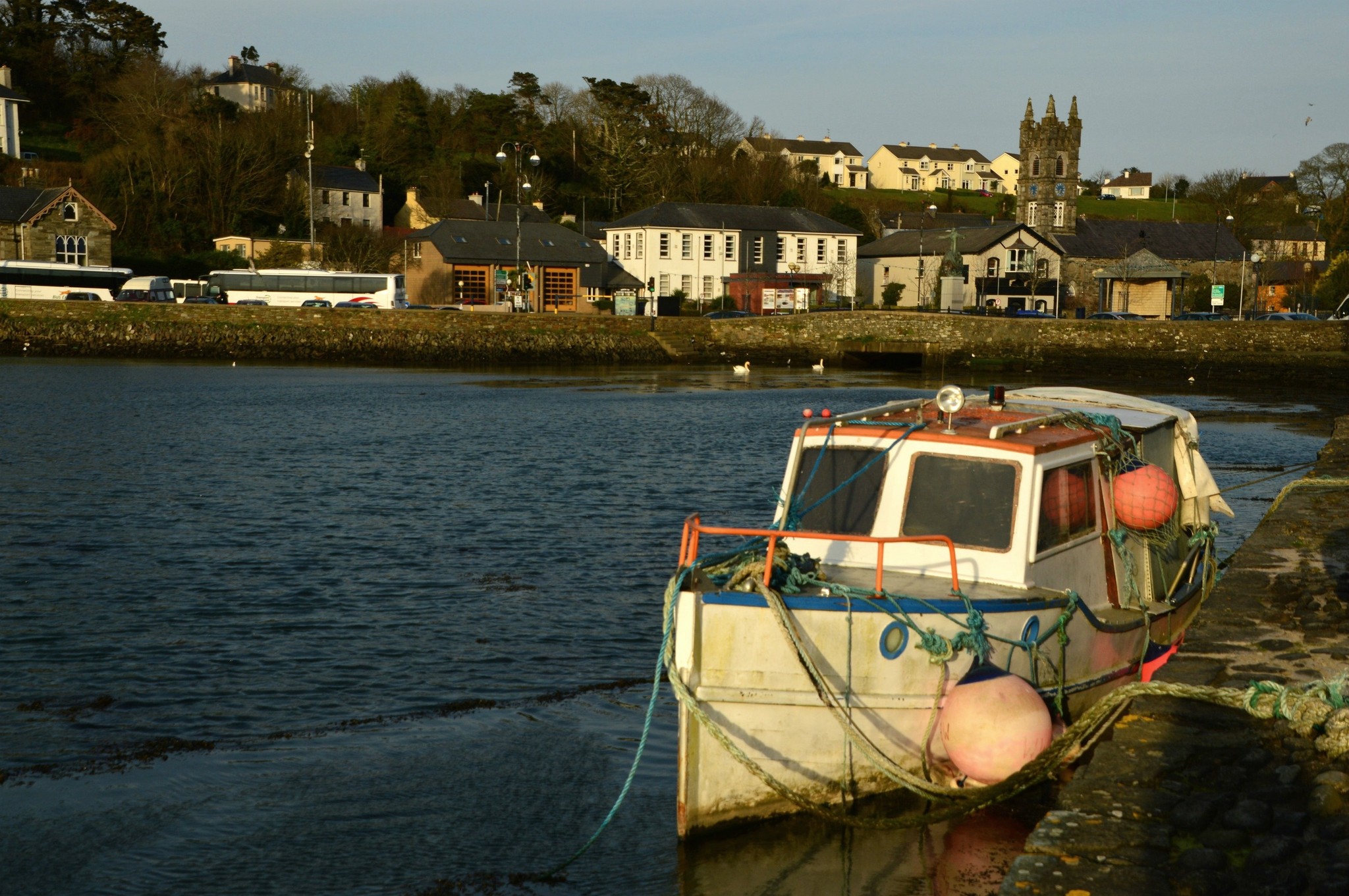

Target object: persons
[216,294,222,304]
[223,294,228,304]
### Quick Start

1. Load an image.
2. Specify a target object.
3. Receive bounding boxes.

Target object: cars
[1010,310,1055,318]
[1253,312,1320,321]
[974,189,994,197]
[1085,312,1147,320]
[1171,312,1232,321]
[1096,194,1116,200]
[170,279,462,310]
[703,311,758,319]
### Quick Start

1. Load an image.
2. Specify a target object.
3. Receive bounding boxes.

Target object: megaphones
[305,139,314,144]
[305,152,311,158]
[307,144,314,150]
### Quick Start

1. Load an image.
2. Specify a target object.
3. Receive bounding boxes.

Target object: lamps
[937,385,965,435]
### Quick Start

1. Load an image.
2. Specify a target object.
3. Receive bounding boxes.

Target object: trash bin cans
[1076,307,1085,319]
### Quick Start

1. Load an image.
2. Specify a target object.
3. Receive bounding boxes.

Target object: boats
[672,386,1234,839]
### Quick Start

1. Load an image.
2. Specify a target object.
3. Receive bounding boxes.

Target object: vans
[1326,294,1349,320]
[114,276,176,303]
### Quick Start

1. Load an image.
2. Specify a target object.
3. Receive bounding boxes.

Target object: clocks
[1028,183,1038,197]
[1055,183,1065,196]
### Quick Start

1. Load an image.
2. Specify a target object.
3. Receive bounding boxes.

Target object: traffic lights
[647,277,654,292]
[524,274,531,290]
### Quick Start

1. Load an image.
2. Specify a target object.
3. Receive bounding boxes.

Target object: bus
[0,260,133,302]
[198,269,405,309]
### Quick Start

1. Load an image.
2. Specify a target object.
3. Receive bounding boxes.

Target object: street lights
[917,197,937,312]
[1250,249,1266,320]
[495,142,540,312]
[1212,209,1234,313]
[784,262,800,289]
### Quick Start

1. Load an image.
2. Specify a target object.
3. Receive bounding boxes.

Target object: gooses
[812,359,824,370]
[733,361,750,374]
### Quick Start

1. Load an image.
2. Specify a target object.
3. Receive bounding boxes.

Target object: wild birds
[232,362,235,367]
